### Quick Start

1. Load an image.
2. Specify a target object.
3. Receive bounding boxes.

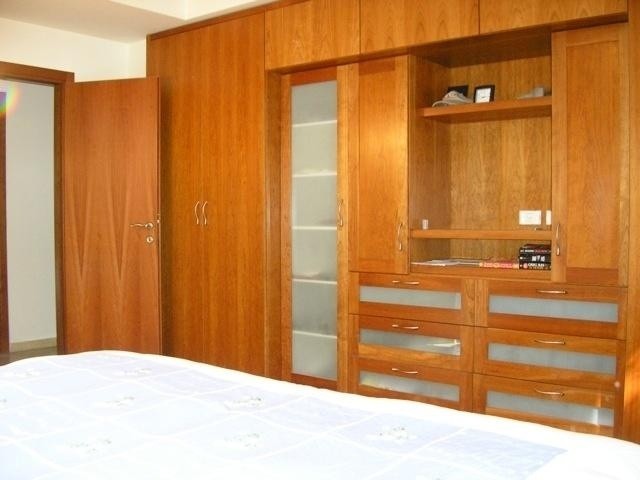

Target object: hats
[431,90,473,107]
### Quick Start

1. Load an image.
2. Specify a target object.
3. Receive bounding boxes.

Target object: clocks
[473,84,494,105]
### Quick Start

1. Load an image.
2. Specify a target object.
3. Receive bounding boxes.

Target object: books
[410,258,479,267]
[479,260,519,270]
[518,253,551,263]
[519,262,551,270]
[519,244,551,255]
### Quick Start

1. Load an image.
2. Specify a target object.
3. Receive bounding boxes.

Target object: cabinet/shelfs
[346,56,410,272]
[265,3,358,72]
[410,35,552,272]
[271,68,335,386]
[359,2,481,56]
[551,21,633,284]
[480,0,627,30]
[151,9,267,374]
[473,275,629,439]
[349,275,471,410]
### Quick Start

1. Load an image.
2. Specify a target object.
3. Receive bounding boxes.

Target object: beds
[2,345,640,449]
